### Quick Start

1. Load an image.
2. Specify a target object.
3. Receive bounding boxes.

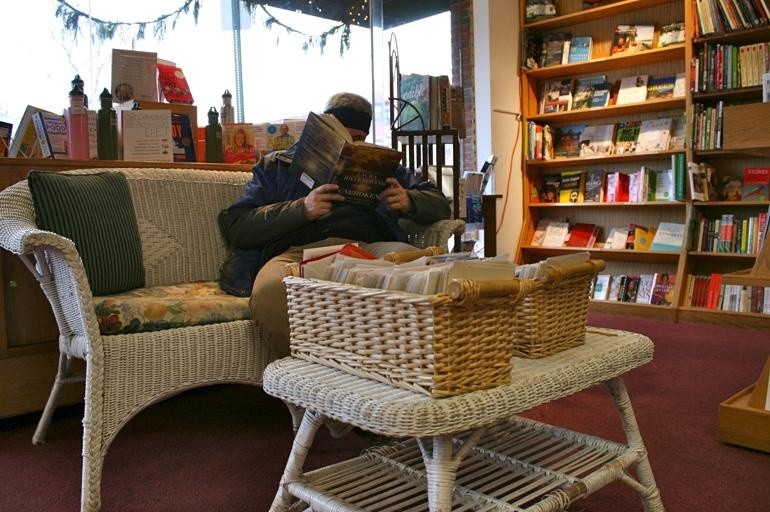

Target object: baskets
[282,263,536,398]
[385,246,606,360]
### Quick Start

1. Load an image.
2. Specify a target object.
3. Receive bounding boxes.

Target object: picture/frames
[137,100,199,161]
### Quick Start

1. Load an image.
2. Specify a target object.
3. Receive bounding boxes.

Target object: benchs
[1,168,465,512]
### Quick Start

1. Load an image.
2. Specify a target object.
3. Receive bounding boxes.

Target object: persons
[216,91,453,450]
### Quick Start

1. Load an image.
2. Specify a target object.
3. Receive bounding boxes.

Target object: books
[222,119,307,164]
[524,0,769,318]
[290,111,405,214]
[448,153,498,256]
[399,73,467,139]
[286,242,593,295]
[110,50,196,164]
[2,104,98,160]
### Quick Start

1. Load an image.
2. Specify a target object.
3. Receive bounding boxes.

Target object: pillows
[28,168,145,296]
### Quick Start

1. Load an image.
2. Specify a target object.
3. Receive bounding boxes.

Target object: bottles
[72,76,88,107]
[205,106,224,162]
[65,87,90,161]
[96,87,119,160]
[222,89,234,125]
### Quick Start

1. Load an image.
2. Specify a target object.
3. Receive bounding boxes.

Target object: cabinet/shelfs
[513,1,768,329]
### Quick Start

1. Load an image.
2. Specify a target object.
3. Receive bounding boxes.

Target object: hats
[324,93,372,133]
